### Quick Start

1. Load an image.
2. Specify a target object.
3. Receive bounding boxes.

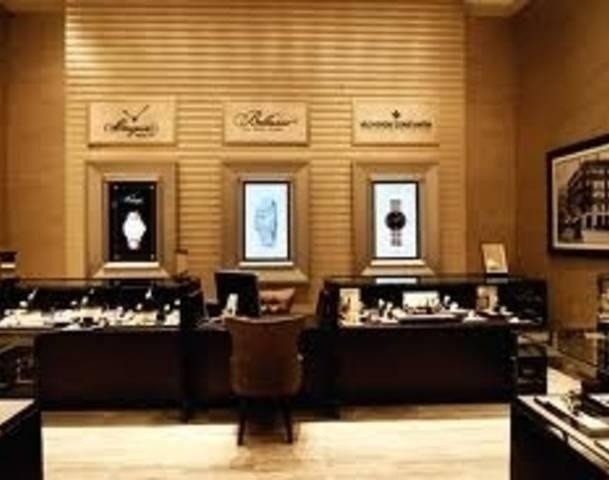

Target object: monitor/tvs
[213,270,259,326]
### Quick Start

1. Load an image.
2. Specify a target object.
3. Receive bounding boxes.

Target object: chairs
[223,314,308,444]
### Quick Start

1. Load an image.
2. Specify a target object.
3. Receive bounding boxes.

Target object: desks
[1,306,557,410]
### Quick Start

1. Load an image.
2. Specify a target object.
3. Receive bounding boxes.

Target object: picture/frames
[100,173,164,268]
[237,175,295,268]
[545,131,609,259]
[364,172,426,266]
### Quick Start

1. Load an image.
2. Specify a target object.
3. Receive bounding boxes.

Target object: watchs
[386,199,406,246]
[122,212,146,250]
[253,198,277,247]
